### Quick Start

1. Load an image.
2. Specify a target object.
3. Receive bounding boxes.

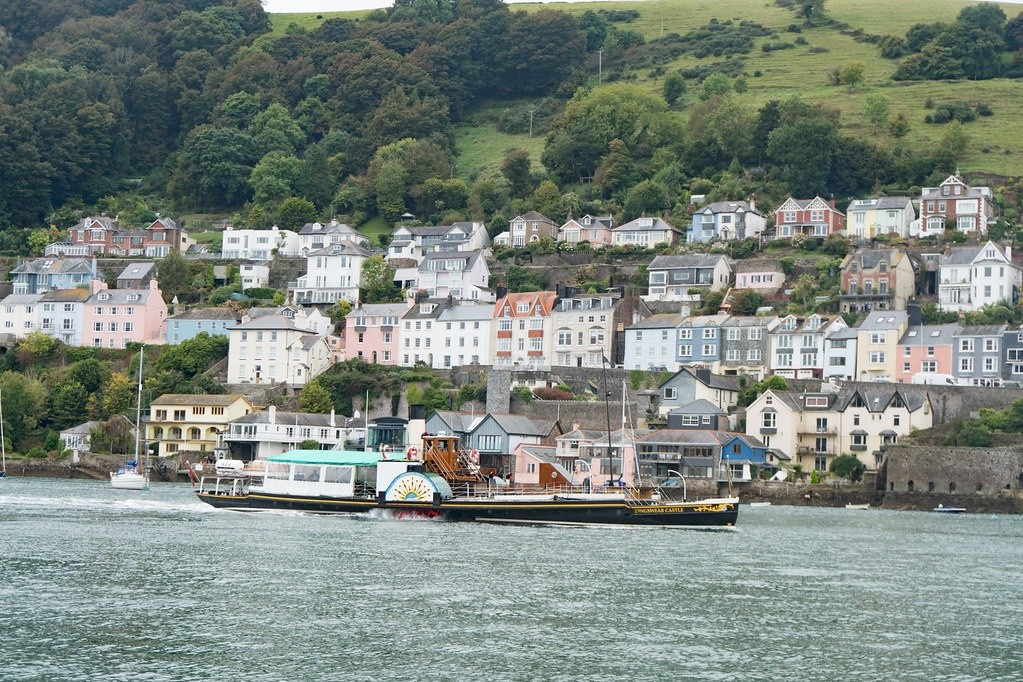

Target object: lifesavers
[471,448,480,463]
[382,444,391,459]
[406,447,418,460]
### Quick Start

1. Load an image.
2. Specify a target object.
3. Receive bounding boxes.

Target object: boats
[194,347,740,526]
[933,507,966,512]
[846,503,869,510]
[108,345,152,489]
